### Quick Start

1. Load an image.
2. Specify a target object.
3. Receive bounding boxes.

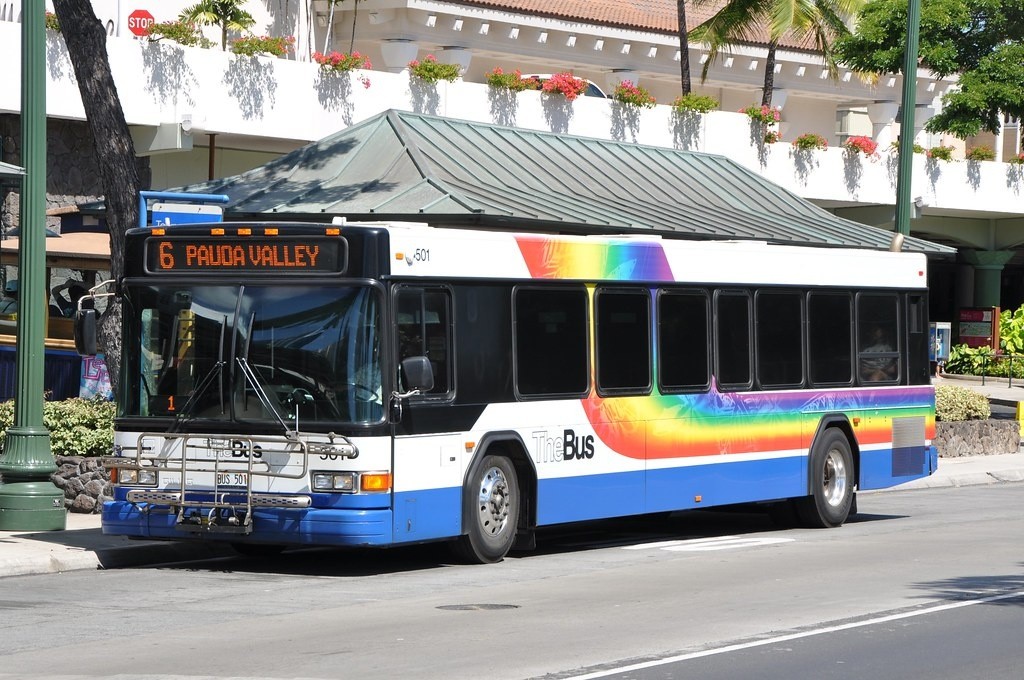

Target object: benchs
[0,320,17,336]
[48,317,75,339]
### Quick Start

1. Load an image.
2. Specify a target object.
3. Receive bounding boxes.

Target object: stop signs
[128,9,154,36]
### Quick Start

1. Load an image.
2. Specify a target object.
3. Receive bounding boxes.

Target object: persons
[860,326,897,381]
[337,359,406,405]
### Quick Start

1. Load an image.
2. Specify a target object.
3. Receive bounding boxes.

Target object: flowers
[964,145,996,161]
[312,51,372,89]
[540,71,590,102]
[146,20,202,47]
[843,135,878,158]
[229,36,296,56]
[738,102,782,126]
[484,66,540,93]
[1008,153,1024,165]
[406,54,461,84]
[613,80,656,109]
[764,130,783,144]
[792,132,829,151]
[926,145,956,163]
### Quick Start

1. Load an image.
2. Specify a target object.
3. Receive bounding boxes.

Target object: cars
[522,74,614,99]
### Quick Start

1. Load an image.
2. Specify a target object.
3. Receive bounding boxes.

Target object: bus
[75,217,938,563]
